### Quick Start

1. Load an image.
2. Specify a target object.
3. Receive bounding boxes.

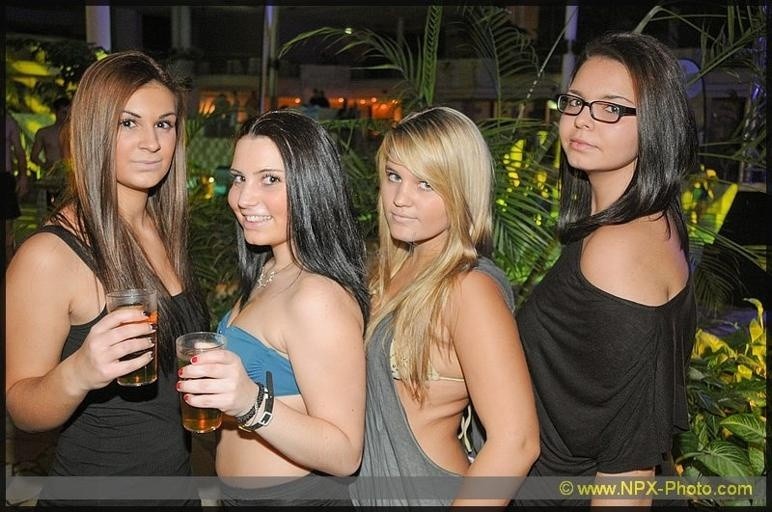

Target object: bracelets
[234,382,264,426]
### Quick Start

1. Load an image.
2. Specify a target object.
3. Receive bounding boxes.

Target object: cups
[174,331,227,434]
[104,288,159,387]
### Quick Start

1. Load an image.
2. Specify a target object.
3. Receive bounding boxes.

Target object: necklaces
[256,248,301,291]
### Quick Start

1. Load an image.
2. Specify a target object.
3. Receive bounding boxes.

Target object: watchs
[238,371,275,434]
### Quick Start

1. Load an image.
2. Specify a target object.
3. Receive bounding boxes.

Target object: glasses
[556,93,638,125]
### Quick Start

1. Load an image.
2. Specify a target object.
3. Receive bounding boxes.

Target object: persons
[245,90,260,118]
[353,100,361,118]
[175,108,367,512]
[0,107,29,265]
[28,98,73,214]
[0,49,207,512]
[231,89,241,109]
[336,100,356,119]
[515,31,699,512]
[346,105,541,512]
[215,93,230,111]
[309,88,330,108]
